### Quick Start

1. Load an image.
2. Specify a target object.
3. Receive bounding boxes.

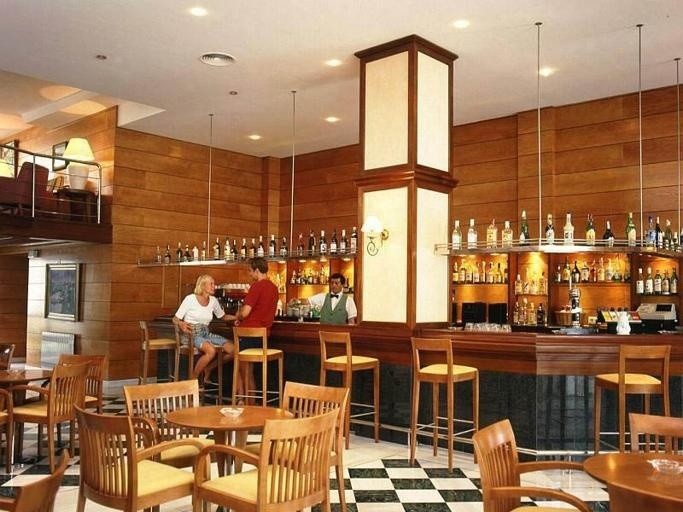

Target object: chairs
[0,162,50,217]
[35,176,73,220]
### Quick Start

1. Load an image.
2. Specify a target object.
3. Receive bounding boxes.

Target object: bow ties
[330,294,339,299]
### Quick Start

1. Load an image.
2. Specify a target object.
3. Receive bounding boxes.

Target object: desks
[51,188,96,224]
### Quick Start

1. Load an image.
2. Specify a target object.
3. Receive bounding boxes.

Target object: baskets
[553,310,586,326]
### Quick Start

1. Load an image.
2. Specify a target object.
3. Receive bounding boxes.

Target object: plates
[220,407,244,417]
[6,369,25,375]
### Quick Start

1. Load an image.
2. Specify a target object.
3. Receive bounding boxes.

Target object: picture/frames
[1,139,20,177]
[43,262,83,323]
[51,140,70,172]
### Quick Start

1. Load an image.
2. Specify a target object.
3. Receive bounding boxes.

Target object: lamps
[60,136,95,190]
[358,214,389,256]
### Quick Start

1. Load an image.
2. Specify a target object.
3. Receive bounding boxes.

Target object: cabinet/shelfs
[448,245,683,329]
[260,253,353,314]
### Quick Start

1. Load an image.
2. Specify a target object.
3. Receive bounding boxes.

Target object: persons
[233,256,280,406]
[286,272,357,326]
[171,274,239,391]
[60,279,73,315]
[48,287,63,314]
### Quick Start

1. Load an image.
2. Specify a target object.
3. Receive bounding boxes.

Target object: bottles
[318,230,327,256]
[163,244,171,264]
[518,209,529,246]
[584,213,595,246]
[451,220,462,251]
[613,255,622,283]
[154,244,161,263]
[661,269,670,295]
[339,229,346,254]
[290,265,326,284]
[571,260,580,283]
[223,237,230,260]
[214,237,220,260]
[644,266,654,296]
[451,258,509,283]
[605,257,613,283]
[279,235,288,257]
[466,219,477,250]
[622,256,631,283]
[624,212,636,247]
[580,260,588,283]
[670,266,678,295]
[176,240,182,263]
[635,267,644,296]
[240,238,246,261]
[329,231,337,255]
[514,271,548,295]
[200,240,207,261]
[248,238,256,260]
[268,234,276,258]
[643,216,679,252]
[653,269,661,296]
[182,240,190,262]
[544,213,554,245]
[501,220,513,249]
[191,239,198,261]
[562,256,571,283]
[536,302,545,325]
[308,232,316,257]
[588,258,596,283]
[256,235,264,258]
[554,265,561,283]
[231,240,238,260]
[563,213,574,247]
[596,257,605,283]
[296,232,304,257]
[350,227,358,254]
[486,218,497,249]
[602,220,615,248]
[514,298,534,324]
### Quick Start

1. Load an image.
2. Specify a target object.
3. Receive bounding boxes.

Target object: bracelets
[296,299,302,305]
